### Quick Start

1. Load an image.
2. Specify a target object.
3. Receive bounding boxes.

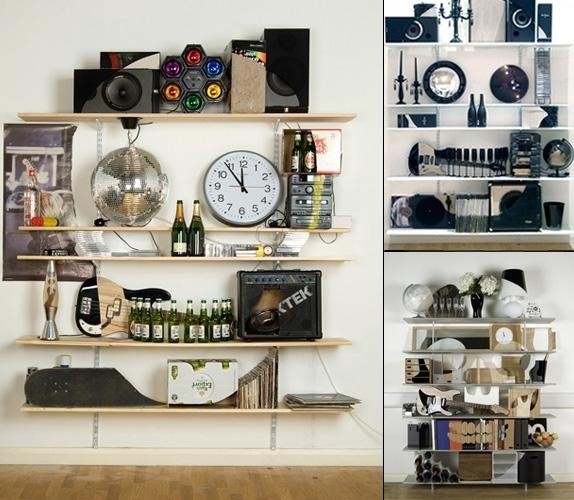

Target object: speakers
[73,68,159,113]
[260,29,310,113]
[385,17,438,43]
[237,269,323,340]
[505,0,535,43]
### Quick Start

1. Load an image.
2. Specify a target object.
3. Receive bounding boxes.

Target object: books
[283,393,362,411]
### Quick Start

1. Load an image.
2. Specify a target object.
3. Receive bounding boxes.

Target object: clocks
[202,150,284,227]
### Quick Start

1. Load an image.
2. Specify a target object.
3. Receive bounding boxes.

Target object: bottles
[188,200,204,256]
[304,130,316,172]
[127,296,235,343]
[290,130,304,172]
[23,169,39,224]
[170,200,188,257]
[478,93,486,127]
[30,216,58,225]
[466,93,476,126]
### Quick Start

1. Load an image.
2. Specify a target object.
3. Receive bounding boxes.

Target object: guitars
[408,143,504,175]
[75,277,237,338]
[417,387,510,416]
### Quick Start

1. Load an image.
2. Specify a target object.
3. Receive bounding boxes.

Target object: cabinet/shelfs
[14,111,358,418]
[402,316,557,489]
[384,41,571,243]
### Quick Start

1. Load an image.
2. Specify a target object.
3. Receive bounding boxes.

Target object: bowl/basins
[534,438,554,447]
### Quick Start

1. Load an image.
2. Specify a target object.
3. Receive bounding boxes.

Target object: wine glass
[443,147,509,178]
[432,296,464,318]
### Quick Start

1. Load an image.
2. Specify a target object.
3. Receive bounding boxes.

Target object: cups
[543,201,564,230]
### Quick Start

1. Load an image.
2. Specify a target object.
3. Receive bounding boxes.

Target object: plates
[423,61,465,102]
[490,65,529,103]
